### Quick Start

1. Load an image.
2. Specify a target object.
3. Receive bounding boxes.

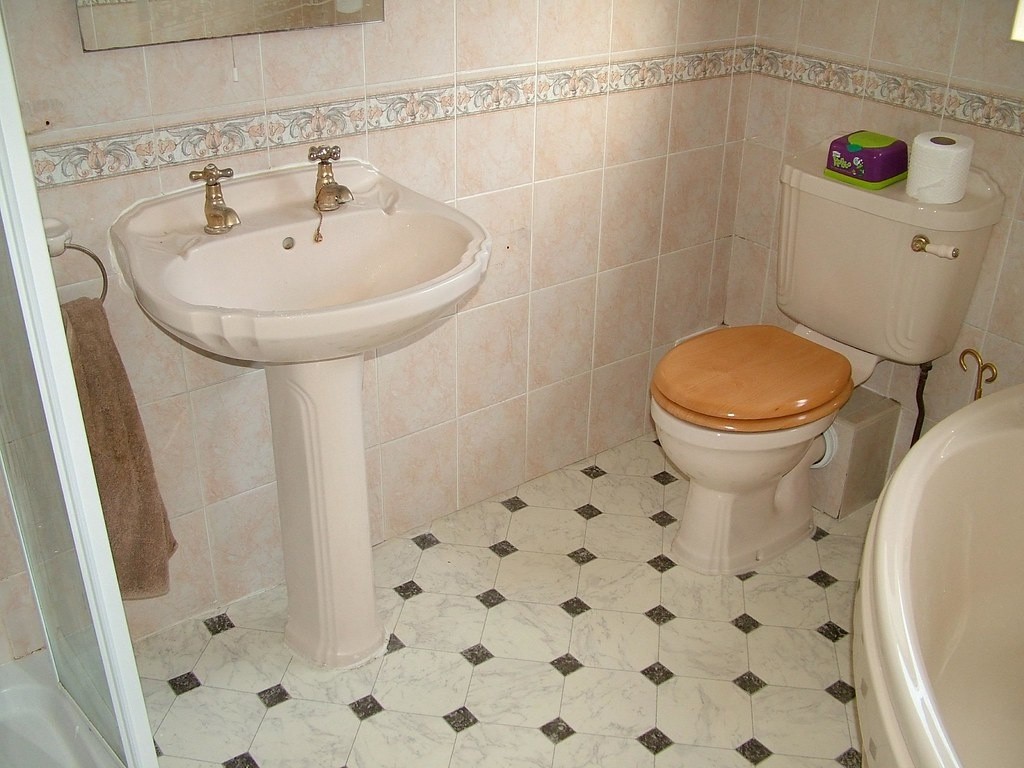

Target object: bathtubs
[846,380,1022,766]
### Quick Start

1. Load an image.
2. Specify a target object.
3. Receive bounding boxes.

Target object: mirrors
[75,0,385,52]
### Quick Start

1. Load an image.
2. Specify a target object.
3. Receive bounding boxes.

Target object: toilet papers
[905,129,975,207]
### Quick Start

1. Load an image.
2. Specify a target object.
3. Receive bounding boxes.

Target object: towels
[61,297,180,603]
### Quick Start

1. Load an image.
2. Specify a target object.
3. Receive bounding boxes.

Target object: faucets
[307,144,355,213]
[188,163,242,236]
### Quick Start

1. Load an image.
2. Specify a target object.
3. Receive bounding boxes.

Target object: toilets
[647,133,1005,578]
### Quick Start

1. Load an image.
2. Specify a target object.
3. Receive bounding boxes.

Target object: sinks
[142,205,489,365]
